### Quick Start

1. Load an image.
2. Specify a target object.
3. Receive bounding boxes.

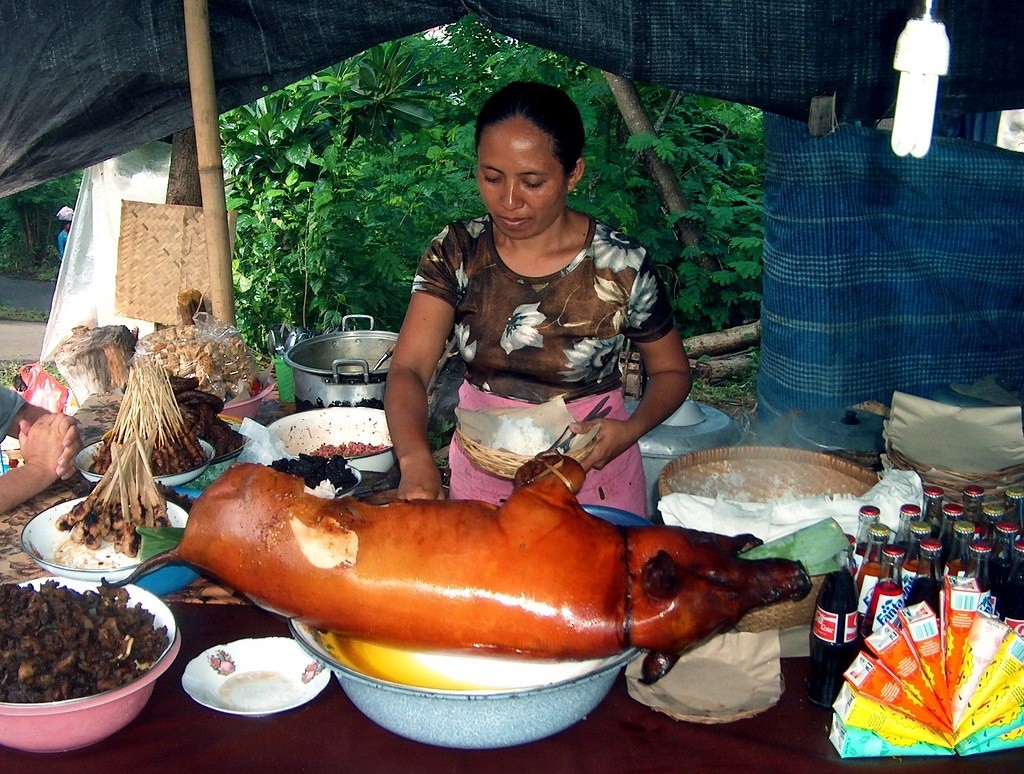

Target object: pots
[624,396,746,519]
[281,313,401,412]
[785,407,886,465]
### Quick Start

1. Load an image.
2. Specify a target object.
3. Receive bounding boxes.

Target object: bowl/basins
[334,463,364,499]
[286,615,647,749]
[73,437,216,487]
[20,495,191,578]
[219,376,277,421]
[661,397,708,427]
[266,405,399,474]
[0,576,182,753]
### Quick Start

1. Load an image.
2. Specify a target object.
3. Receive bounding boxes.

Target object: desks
[0,601,1024,774]
[0,393,293,605]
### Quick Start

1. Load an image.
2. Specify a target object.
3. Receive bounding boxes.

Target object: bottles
[804,486,1024,709]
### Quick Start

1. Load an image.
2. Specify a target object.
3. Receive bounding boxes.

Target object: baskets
[658,445,884,633]
[885,440,1024,519]
[455,408,598,479]
[652,672,785,724]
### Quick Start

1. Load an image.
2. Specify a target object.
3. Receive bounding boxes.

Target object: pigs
[99,461,815,685]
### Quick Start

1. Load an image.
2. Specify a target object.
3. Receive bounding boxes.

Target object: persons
[58,206,73,260]
[0,382,84,517]
[384,82,692,519]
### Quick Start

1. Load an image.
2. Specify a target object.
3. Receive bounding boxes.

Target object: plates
[181,635,332,717]
